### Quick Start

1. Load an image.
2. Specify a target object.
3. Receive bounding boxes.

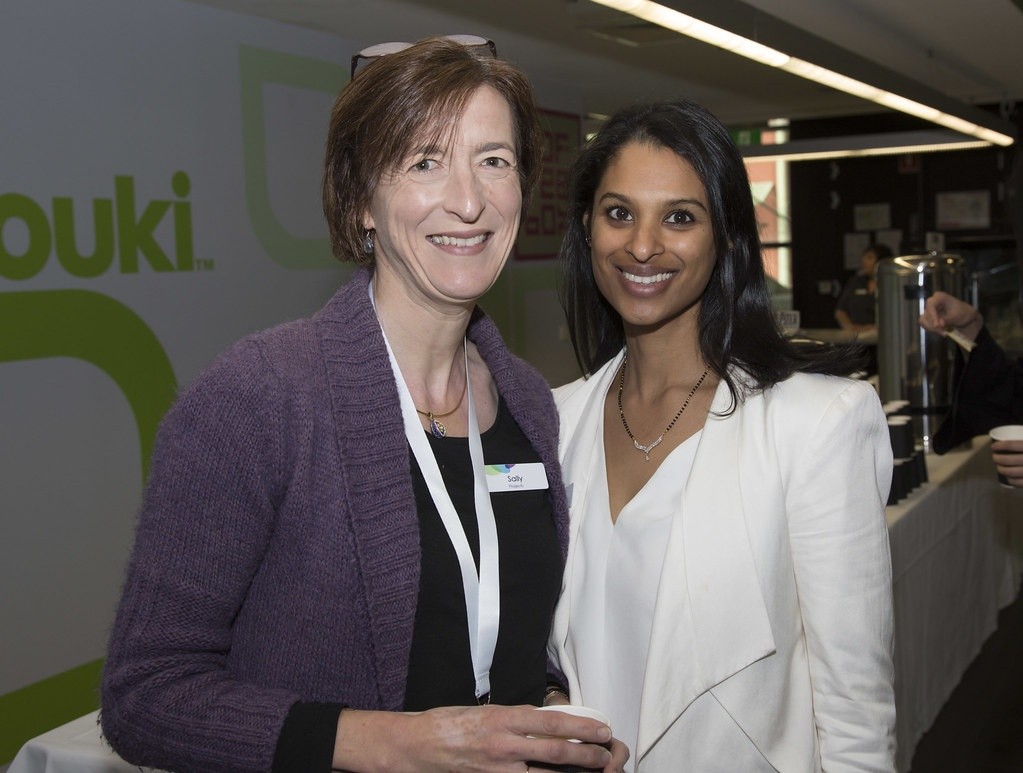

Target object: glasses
[349,36,496,81]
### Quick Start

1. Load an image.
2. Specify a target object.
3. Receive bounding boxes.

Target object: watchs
[544,688,568,704]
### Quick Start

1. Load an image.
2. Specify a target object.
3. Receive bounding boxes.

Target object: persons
[99,36,629,773]
[915,292,1023,488]
[539,97,899,773]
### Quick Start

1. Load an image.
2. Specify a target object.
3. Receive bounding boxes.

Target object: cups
[524,704,610,773]
[880,398,928,507]
[989,425,1023,489]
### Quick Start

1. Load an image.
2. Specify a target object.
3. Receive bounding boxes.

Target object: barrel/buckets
[873,253,977,411]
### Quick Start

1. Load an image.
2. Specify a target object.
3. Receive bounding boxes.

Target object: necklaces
[415,372,467,438]
[618,355,710,462]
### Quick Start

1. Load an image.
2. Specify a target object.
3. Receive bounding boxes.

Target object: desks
[7,438,993,773]
